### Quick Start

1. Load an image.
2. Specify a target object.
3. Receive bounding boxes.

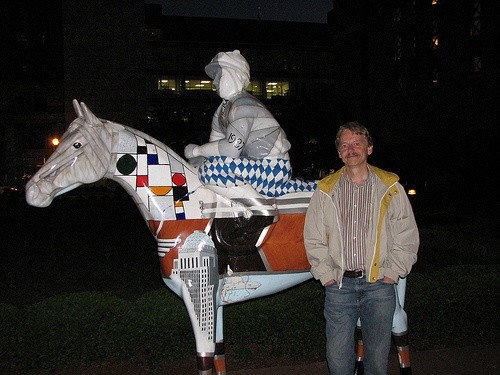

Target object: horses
[23,97,413,375]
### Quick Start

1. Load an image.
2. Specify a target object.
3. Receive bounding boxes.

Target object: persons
[302,121,421,375]
[184,49,292,239]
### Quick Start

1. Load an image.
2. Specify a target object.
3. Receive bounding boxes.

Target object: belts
[343,269,366,279]
